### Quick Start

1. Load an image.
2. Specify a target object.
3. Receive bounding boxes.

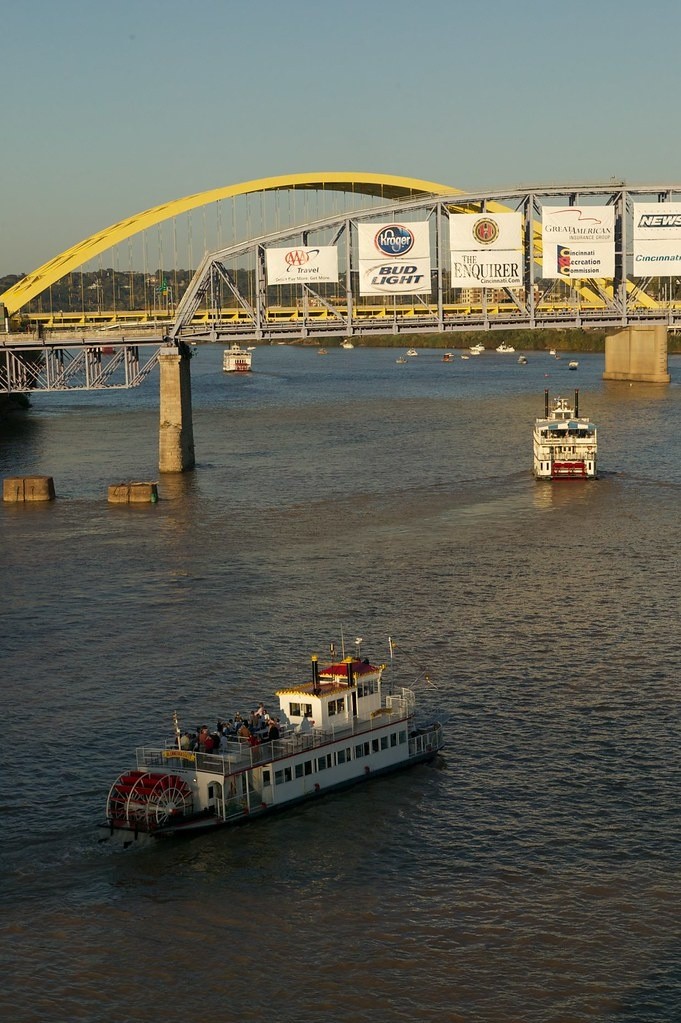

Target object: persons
[175,703,281,753]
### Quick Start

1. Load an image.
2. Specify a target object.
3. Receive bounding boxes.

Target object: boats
[469,342,485,355]
[246,346,257,351]
[406,348,419,357]
[222,342,252,371]
[461,355,470,360]
[568,360,578,370]
[516,353,528,364]
[340,340,354,349]
[95,622,448,850]
[550,348,556,355]
[441,352,454,362]
[318,348,328,354]
[496,340,515,353]
[396,357,408,364]
[533,387,599,483]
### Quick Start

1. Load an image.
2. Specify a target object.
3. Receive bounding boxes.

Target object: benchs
[266,734,323,753]
